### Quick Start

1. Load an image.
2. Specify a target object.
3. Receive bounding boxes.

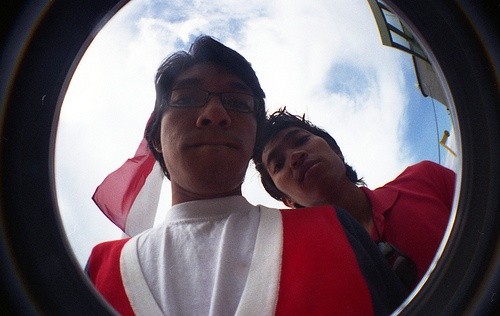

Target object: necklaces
[360,187,400,255]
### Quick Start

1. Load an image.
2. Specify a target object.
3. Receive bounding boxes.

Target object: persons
[254,108,456,295]
[84,34,406,316]
